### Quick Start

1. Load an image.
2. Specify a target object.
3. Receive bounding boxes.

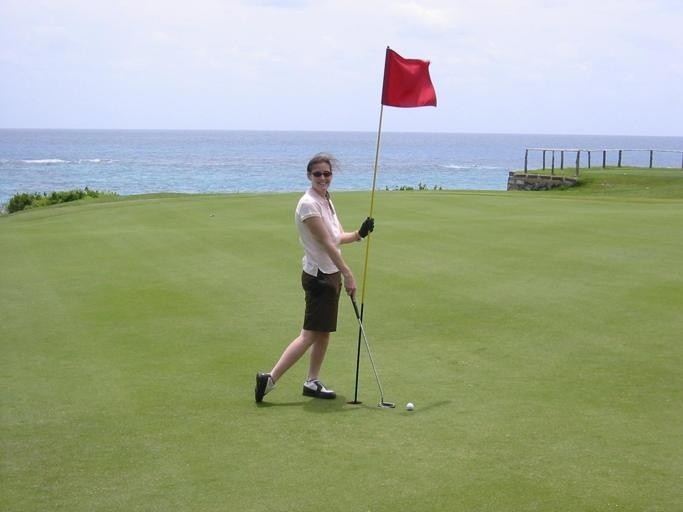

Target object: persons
[251,149,374,404]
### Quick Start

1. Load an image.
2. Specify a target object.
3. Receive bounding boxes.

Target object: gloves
[358,217,374,238]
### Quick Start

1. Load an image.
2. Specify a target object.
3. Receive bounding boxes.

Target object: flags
[383,46,437,107]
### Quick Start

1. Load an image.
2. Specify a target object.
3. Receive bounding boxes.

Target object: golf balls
[405,402,415,411]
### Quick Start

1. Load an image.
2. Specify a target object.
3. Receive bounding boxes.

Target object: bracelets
[354,231,361,242]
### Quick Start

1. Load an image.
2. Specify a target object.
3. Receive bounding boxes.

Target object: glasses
[312,171,332,178]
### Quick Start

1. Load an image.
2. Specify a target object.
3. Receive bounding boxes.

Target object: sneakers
[254,372,274,403]
[303,378,336,399]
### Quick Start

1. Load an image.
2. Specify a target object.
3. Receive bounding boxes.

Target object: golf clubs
[348,291,396,409]
